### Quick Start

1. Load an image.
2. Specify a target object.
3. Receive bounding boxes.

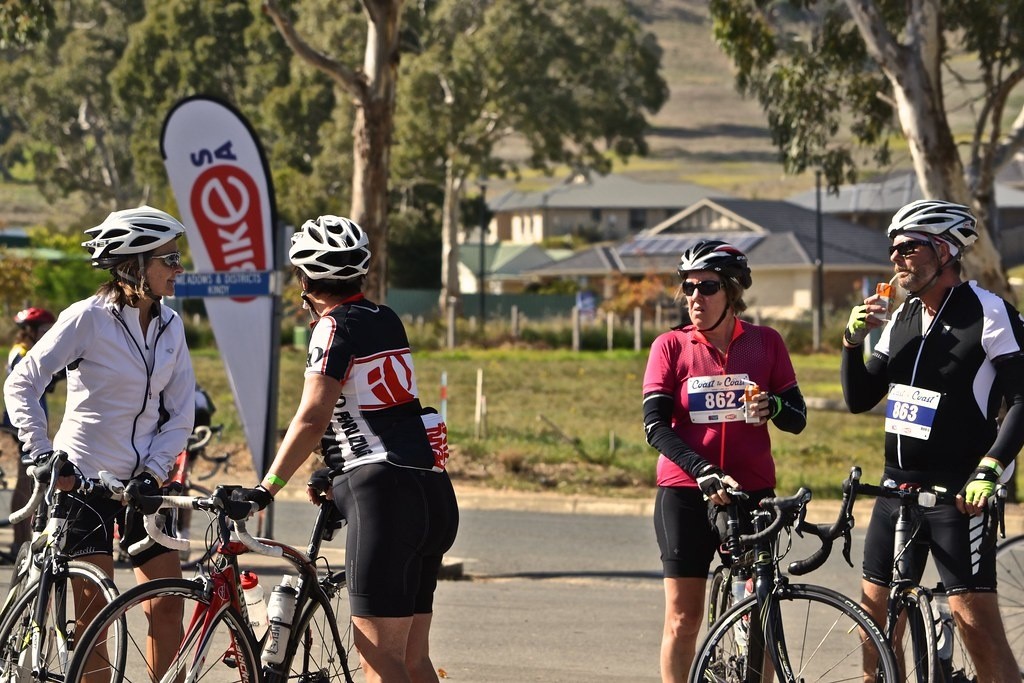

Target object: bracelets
[643,239,806,683]
[979,460,1003,476]
[265,473,287,488]
[771,396,782,420]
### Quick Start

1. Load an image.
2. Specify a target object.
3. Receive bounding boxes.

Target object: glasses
[681,281,725,297]
[151,252,181,267]
[888,240,932,256]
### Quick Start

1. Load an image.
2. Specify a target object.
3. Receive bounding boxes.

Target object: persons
[4,309,67,557]
[212,213,460,683]
[178,385,212,563]
[841,200,1024,683]
[6,206,194,683]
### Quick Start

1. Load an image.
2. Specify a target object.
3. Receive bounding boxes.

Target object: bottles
[261,575,299,665]
[24,552,44,590]
[930,581,954,659]
[239,569,273,650]
[732,569,749,647]
[741,577,754,641]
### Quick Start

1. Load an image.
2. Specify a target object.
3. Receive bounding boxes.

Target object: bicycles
[843,468,1024,683]
[66,469,368,683]
[685,484,899,683]
[0,448,165,683]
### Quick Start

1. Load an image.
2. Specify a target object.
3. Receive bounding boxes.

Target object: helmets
[14,307,56,327]
[677,240,752,289]
[888,199,979,253]
[81,206,186,269]
[289,215,371,279]
